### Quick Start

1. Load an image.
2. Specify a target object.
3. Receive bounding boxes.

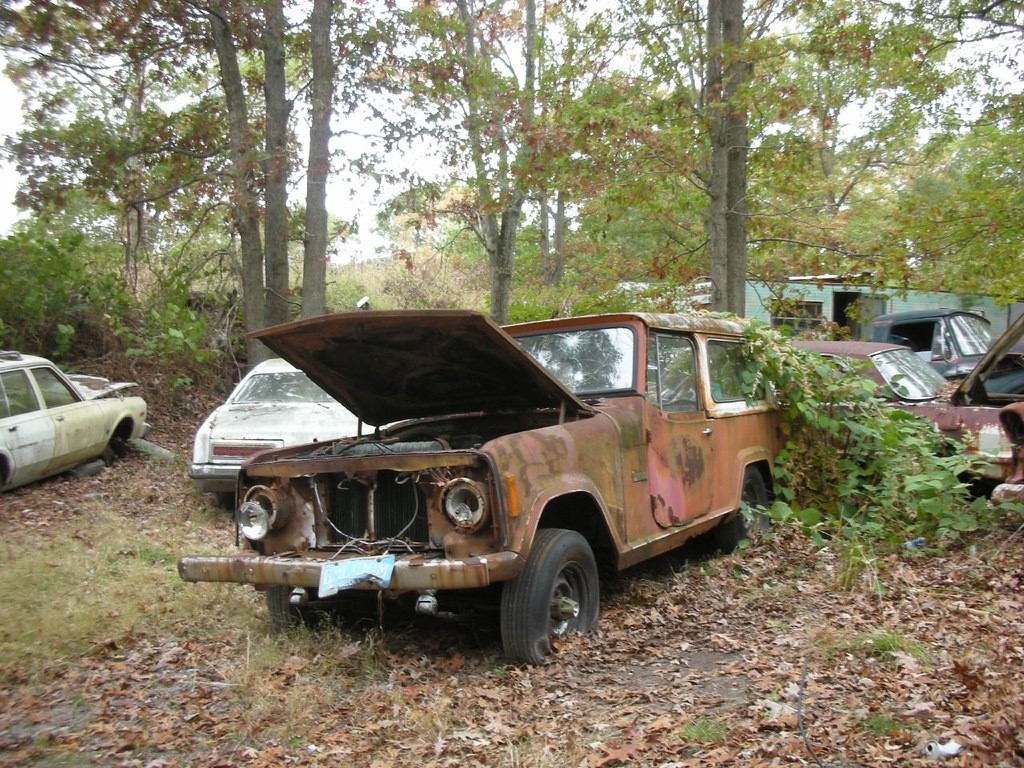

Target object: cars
[782,313,1024,501]
[186,305,775,666]
[867,307,1023,397]
[0,349,147,494]
[183,360,379,508]
